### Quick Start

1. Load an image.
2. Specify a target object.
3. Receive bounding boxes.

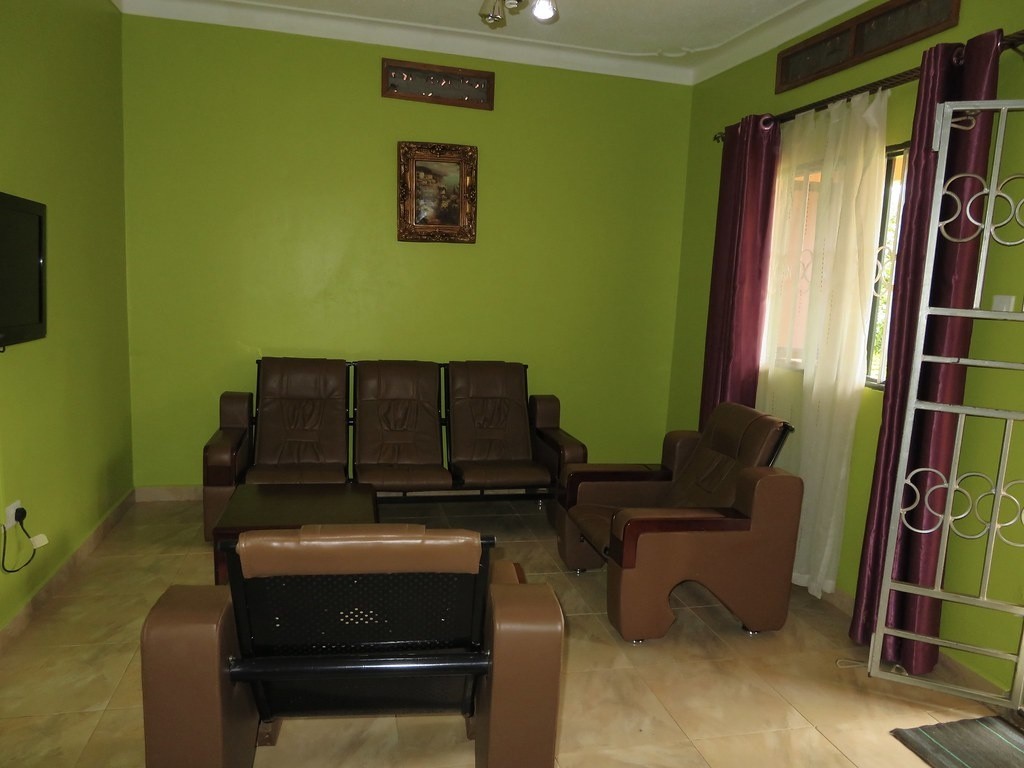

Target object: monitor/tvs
[0,192,47,347]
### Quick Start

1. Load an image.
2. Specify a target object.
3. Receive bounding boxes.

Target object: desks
[212,484,378,588]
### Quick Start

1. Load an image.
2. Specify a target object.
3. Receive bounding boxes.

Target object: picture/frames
[397,141,478,243]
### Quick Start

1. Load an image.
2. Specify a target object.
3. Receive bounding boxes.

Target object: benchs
[204,356,587,542]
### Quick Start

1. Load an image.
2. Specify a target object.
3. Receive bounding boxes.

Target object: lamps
[479,0,557,24]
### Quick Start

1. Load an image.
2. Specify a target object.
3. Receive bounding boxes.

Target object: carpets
[890,716,1024,768]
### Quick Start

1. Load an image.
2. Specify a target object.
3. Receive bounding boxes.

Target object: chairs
[140,522,565,768]
[556,401,805,644]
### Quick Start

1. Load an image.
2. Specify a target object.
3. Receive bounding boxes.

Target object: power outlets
[6,499,20,528]
